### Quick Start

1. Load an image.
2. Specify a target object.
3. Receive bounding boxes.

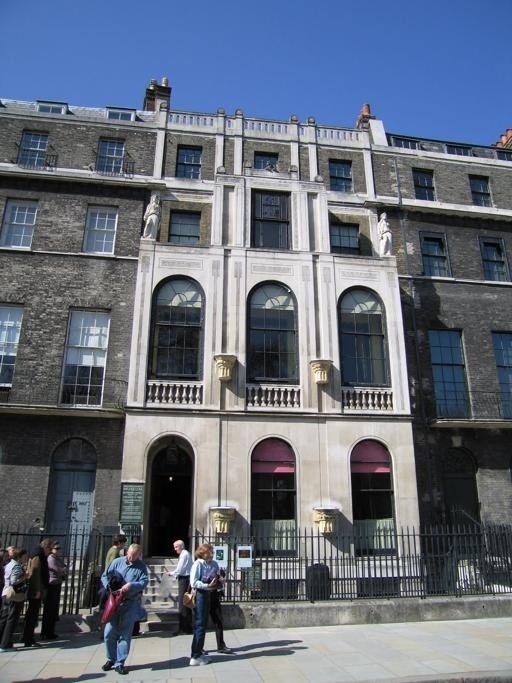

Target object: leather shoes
[172,630,192,635]
[39,634,58,640]
[102,660,114,671]
[115,665,128,674]
[24,641,41,647]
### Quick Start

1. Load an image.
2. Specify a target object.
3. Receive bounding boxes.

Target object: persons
[0,538,66,652]
[105,535,142,637]
[378,212,392,256]
[143,194,161,239]
[166,539,192,635]
[189,544,232,666]
[100,543,148,674]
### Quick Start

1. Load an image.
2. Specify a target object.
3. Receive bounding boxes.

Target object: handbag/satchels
[6,581,27,602]
[101,588,123,624]
[182,591,195,609]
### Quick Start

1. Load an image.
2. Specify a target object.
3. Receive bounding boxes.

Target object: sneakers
[188,649,212,666]
[0,647,18,652]
[216,647,232,653]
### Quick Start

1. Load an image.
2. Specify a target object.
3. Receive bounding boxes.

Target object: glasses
[51,547,61,550]
